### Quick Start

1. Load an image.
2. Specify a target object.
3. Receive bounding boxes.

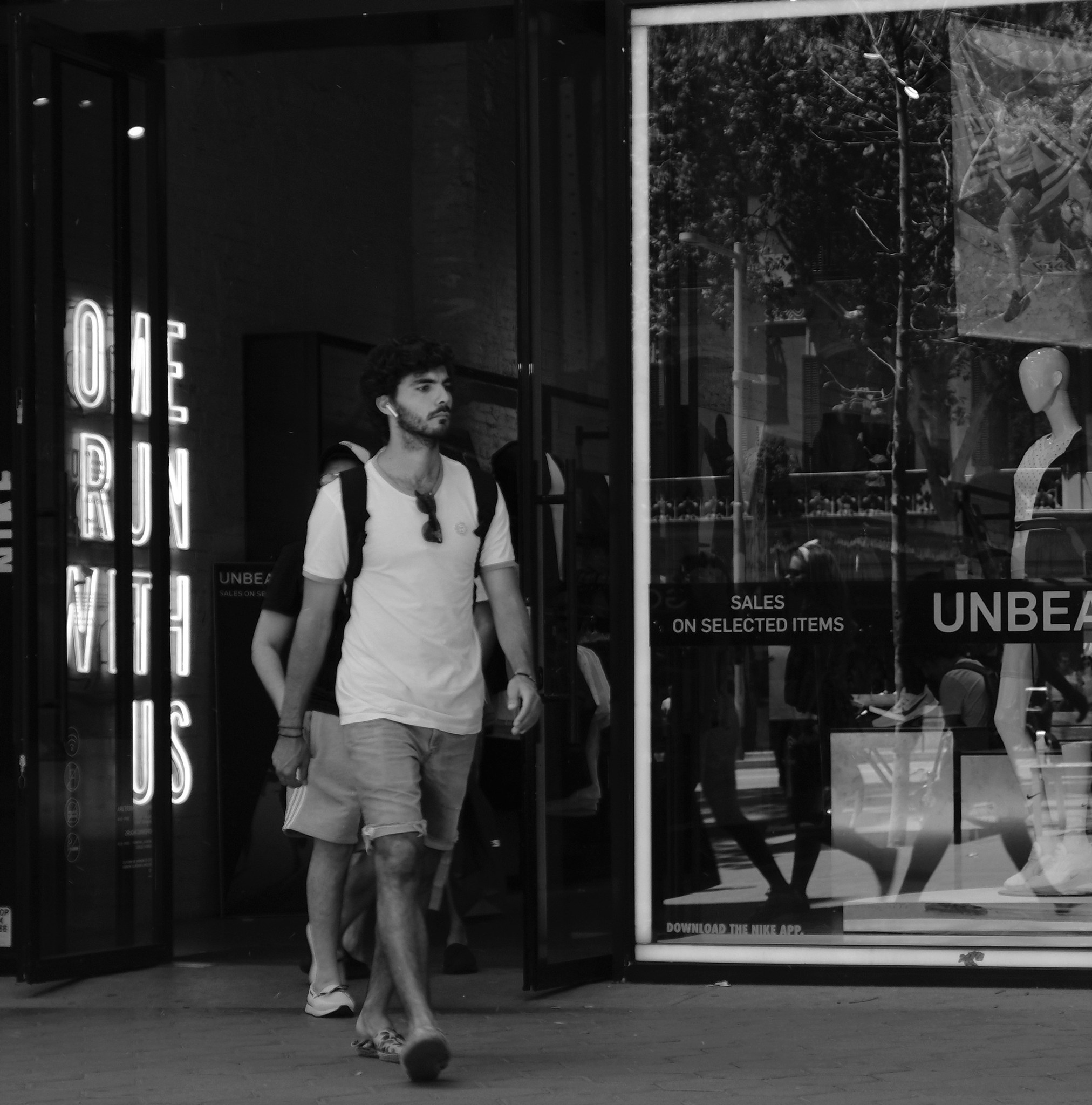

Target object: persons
[269,334,546,1085]
[992,349,1092,902]
[254,440,391,1018]
[650,538,1082,925]
[301,585,491,977]
[977,92,1075,327]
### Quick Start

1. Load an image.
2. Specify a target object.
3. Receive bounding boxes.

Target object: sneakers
[874,688,939,731]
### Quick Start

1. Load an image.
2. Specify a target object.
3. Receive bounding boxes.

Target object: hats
[315,440,373,469]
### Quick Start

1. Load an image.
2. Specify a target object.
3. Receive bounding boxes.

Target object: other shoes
[442,942,477,973]
[300,942,371,980]
[352,1028,404,1060]
[307,923,347,988]
[402,1025,452,1082]
[305,982,355,1018]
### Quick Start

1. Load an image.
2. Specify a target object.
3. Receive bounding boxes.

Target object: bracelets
[277,725,304,730]
[510,671,537,683]
[278,732,304,738]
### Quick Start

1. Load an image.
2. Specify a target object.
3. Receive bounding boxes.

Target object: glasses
[414,489,442,542]
[321,474,339,486]
[786,568,804,577]
